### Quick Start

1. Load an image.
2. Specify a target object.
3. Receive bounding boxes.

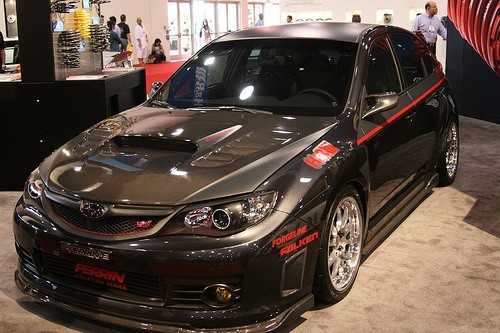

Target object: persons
[151,38,166,64]
[412,1,448,57]
[255,13,264,26]
[168,21,176,36]
[182,21,188,36]
[100,14,133,52]
[198,18,212,48]
[135,16,149,67]
[0,31,6,74]
[286,15,293,23]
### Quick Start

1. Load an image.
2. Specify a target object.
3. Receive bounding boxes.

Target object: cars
[11,21,461,333]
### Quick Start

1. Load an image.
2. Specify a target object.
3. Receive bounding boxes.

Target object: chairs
[335,57,384,93]
[257,56,296,100]
